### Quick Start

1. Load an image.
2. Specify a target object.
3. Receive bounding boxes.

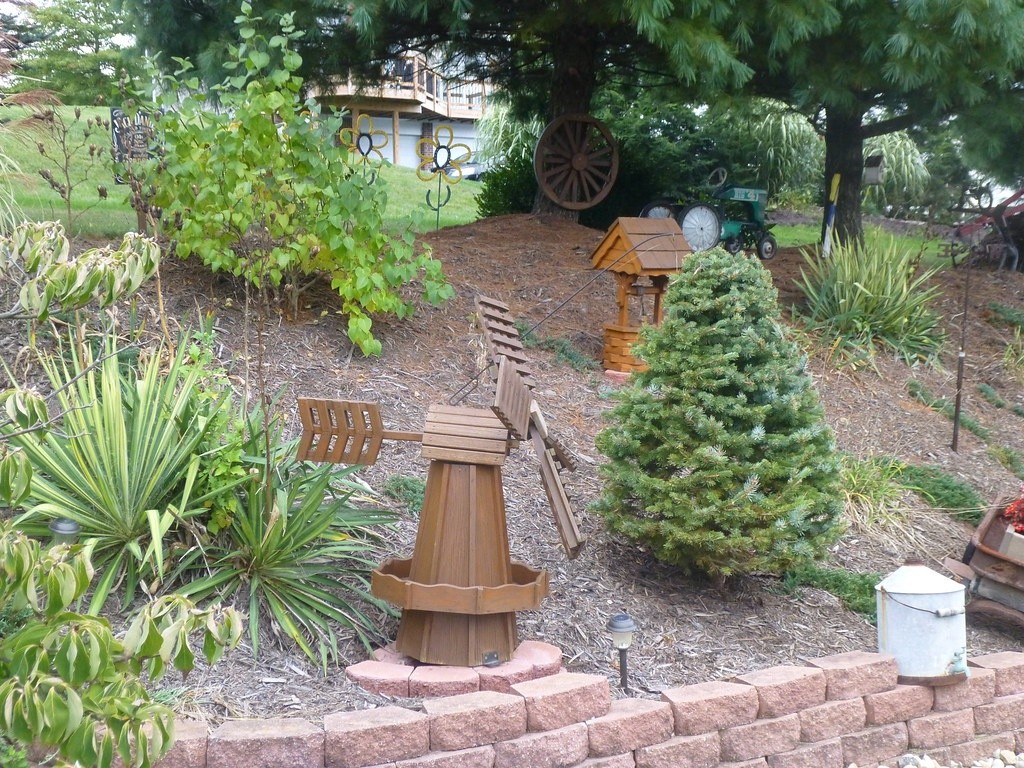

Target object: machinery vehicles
[639,165,777,260]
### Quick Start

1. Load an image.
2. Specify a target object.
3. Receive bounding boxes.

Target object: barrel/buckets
[874,557,967,686]
